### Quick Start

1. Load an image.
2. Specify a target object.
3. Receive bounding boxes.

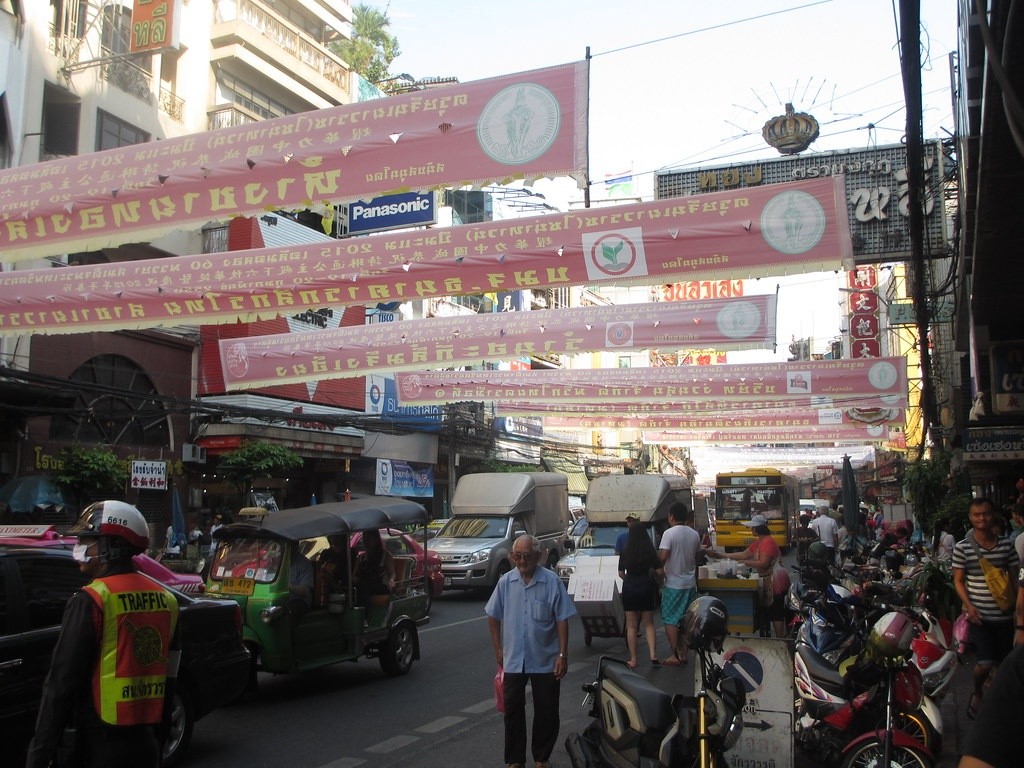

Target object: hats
[625,512,640,520]
[214,514,222,521]
[744,515,768,527]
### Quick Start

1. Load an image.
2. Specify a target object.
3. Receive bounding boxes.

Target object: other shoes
[509,763,525,768]
[760,628,771,637]
[536,761,551,768]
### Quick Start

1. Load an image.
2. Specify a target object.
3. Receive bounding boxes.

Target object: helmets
[63,500,149,554]
[683,596,728,647]
[808,541,827,559]
[873,611,914,652]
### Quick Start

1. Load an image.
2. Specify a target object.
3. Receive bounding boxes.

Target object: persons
[875,528,907,557]
[932,518,955,580]
[615,512,640,555]
[189,525,203,541]
[952,497,1024,768]
[210,515,226,540]
[25,500,181,768]
[800,503,883,561]
[164,525,174,546]
[657,501,707,663]
[619,522,665,668]
[706,515,790,638]
[485,534,577,768]
[319,528,396,627]
[270,541,314,629]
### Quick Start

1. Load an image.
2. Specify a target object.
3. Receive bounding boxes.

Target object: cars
[0,521,206,598]
[210,525,446,619]
[564,515,587,552]
[0,542,244,768]
[408,518,449,546]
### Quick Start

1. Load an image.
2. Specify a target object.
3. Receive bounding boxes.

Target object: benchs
[391,555,421,604]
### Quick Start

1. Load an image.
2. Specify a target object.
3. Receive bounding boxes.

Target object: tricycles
[201,494,431,693]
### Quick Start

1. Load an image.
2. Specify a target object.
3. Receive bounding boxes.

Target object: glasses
[512,553,537,561]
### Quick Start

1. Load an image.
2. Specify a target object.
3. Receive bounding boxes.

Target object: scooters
[780,514,958,768]
[565,597,748,768]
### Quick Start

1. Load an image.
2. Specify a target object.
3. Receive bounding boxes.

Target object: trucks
[422,471,571,602]
[552,472,698,590]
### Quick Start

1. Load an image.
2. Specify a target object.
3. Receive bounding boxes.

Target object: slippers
[984,674,993,687]
[662,657,687,666]
[652,660,662,666]
[967,692,977,720]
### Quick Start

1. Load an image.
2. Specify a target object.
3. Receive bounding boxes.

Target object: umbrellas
[173,482,186,543]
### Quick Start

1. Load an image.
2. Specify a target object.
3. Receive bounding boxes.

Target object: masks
[71,541,102,563]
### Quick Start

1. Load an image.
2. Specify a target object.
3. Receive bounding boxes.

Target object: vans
[799,500,830,520]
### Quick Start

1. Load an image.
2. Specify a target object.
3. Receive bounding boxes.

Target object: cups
[698,559,746,579]
[749,573,759,578]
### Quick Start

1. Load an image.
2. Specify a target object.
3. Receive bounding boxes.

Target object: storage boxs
[567,556,627,635]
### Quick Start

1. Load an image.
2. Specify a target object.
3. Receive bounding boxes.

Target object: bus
[709,468,801,555]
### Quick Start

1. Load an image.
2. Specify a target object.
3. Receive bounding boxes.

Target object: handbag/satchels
[495,664,505,712]
[983,567,1015,612]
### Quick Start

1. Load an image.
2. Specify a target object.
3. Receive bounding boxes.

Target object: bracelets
[559,653,567,658]
[1016,626,1024,630]
